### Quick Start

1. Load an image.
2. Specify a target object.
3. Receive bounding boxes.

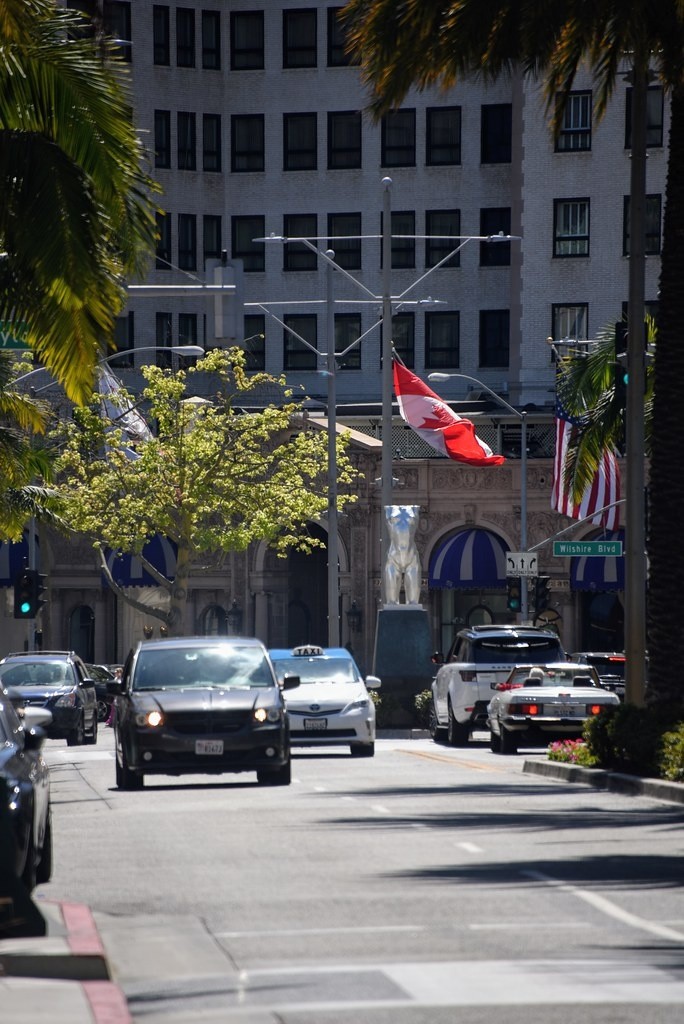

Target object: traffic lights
[13,569,36,619]
[535,576,552,614]
[35,570,48,615]
[506,577,522,613]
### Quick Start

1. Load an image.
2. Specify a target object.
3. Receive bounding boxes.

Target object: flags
[98,372,154,467]
[392,358,507,468]
[550,360,622,533]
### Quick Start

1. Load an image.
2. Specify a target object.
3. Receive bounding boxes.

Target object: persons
[105,667,123,726]
[495,667,544,692]
[53,667,67,681]
[384,505,424,605]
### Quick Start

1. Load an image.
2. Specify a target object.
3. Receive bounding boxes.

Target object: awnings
[429,528,510,590]
[573,532,648,593]
[101,531,178,590]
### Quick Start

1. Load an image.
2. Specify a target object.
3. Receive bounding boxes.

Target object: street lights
[26,346,207,649]
[428,370,530,629]
[250,175,526,660]
[242,297,447,646]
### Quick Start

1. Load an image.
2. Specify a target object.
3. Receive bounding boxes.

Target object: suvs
[568,651,629,702]
[427,624,573,747]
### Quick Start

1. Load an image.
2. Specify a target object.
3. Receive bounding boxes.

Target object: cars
[106,637,301,791]
[0,685,54,933]
[84,663,124,723]
[266,645,383,756]
[485,663,622,755]
[0,649,98,746]
